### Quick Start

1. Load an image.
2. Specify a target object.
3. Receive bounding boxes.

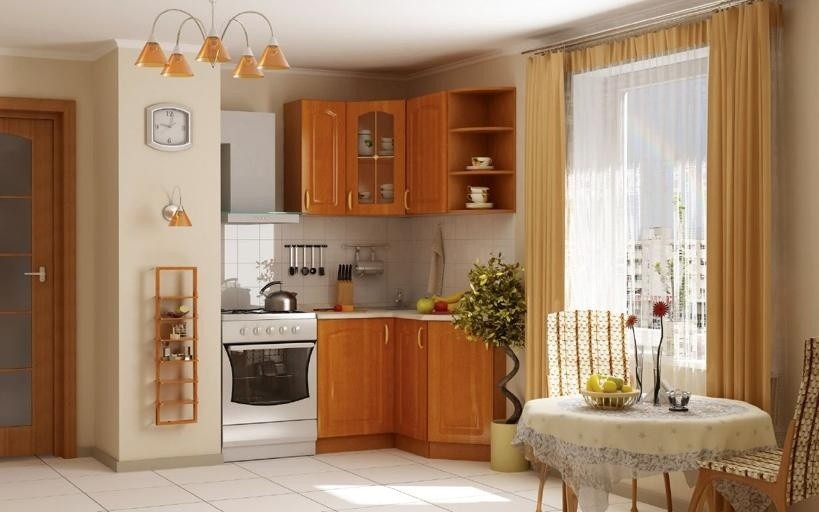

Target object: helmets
[466,203,492,208]
[466,166,494,169]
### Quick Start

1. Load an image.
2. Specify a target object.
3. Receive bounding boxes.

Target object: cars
[468,185,489,202]
[471,158,492,167]
[380,182,395,199]
[381,137,393,150]
[666,391,690,411]
[360,192,371,199]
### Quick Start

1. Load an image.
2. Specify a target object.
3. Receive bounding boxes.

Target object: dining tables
[452,252,534,473]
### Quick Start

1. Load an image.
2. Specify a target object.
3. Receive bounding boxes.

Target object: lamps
[687,338,819,512]
[534,309,675,512]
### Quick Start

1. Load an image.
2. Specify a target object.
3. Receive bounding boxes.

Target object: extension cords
[260,281,297,312]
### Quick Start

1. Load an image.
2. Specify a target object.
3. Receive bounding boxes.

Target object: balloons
[143,101,195,153]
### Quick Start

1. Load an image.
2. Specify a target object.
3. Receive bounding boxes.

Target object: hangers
[289,244,295,275]
[301,244,310,275]
[295,244,299,275]
[310,245,317,274]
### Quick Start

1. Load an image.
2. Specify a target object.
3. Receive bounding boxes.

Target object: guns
[590,375,601,392]
[432,289,466,303]
[587,378,593,391]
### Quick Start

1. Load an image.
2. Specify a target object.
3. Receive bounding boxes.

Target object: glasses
[162,185,192,228]
[135,0,288,80]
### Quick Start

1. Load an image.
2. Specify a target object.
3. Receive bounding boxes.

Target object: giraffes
[223,311,319,459]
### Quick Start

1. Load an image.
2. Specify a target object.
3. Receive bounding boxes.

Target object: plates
[446,85,516,216]
[302,98,344,217]
[393,319,428,456]
[317,318,393,454]
[156,266,199,427]
[406,92,446,216]
[344,101,406,215]
[427,320,493,462]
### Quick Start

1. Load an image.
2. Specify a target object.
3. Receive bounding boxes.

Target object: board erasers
[604,381,617,393]
[621,384,632,392]
[615,378,624,389]
[608,376,615,382]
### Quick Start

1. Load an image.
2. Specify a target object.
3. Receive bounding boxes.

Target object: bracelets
[581,388,640,411]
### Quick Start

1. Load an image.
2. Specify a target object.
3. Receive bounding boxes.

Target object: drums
[338,264,352,281]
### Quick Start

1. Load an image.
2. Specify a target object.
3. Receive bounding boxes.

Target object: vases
[651,301,668,404]
[624,312,644,403]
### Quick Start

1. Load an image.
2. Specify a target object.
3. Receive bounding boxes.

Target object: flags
[652,346,664,407]
[633,345,644,405]
